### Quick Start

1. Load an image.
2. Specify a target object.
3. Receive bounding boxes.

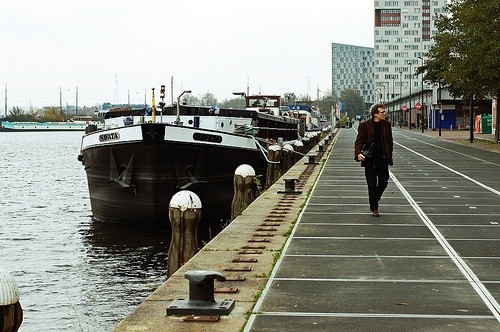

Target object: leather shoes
[373,210,379,216]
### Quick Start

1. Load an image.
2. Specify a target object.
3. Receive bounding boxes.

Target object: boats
[78,77,328,235]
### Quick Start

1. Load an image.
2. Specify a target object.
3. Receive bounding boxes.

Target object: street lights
[379,82,390,122]
[408,61,413,130]
[399,71,402,128]
[392,77,395,127]
[416,56,424,133]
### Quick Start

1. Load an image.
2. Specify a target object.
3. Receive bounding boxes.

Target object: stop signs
[415,103,422,110]
[402,105,407,112]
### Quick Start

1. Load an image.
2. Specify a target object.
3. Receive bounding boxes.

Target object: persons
[412,121,416,128]
[352,117,355,124]
[354,103,393,216]
[359,118,362,122]
[400,116,403,123]
